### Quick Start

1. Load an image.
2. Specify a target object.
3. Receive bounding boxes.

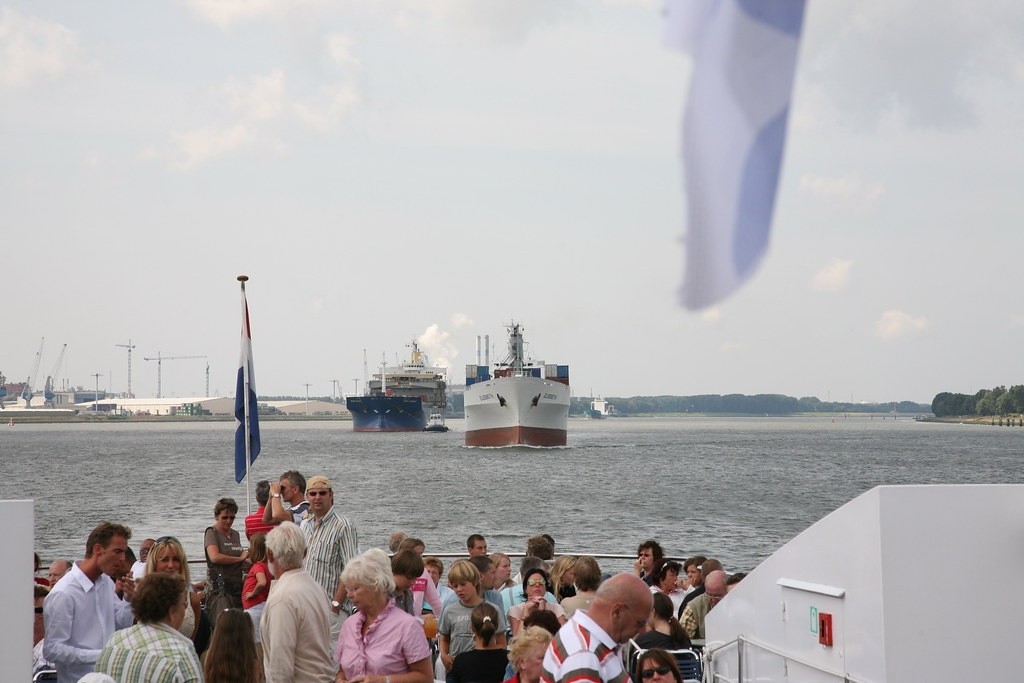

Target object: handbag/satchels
[207,592,243,629]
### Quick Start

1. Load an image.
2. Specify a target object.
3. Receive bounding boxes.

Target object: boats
[346,391,426,432]
[422,406,449,432]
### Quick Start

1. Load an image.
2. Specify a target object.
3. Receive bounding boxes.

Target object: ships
[463,319,570,448]
[364,340,447,422]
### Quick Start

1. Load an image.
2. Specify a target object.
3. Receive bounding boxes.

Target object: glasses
[705,594,723,601]
[219,513,236,520]
[639,664,672,678]
[529,579,547,586]
[308,491,330,496]
[140,548,151,554]
[177,601,189,608]
[659,561,671,572]
[639,553,653,557]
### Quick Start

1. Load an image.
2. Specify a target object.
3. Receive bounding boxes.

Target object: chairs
[628,640,702,682]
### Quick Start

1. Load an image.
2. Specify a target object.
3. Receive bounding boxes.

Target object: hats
[305,475,332,493]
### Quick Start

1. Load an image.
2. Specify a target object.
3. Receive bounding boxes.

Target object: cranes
[26,337,45,391]
[114,339,136,397]
[143,351,207,398]
[48,342,69,388]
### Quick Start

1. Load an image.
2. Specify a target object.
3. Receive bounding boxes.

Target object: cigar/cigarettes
[125,576,135,581]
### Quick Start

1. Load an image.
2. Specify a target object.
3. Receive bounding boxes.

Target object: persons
[35,472,749,683]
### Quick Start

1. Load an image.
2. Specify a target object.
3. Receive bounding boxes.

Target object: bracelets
[385,676,389,683]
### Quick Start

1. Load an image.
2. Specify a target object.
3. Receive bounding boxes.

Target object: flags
[235,296,261,483]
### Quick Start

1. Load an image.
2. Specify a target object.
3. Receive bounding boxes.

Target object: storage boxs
[546,365,570,386]
[466,365,489,386]
[494,368,541,379]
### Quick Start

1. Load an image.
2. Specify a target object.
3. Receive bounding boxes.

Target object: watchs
[271,493,281,498]
[331,601,341,608]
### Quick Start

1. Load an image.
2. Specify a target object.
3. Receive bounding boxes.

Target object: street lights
[91,373,104,412]
[329,380,340,399]
[351,378,361,397]
[302,383,313,415]
